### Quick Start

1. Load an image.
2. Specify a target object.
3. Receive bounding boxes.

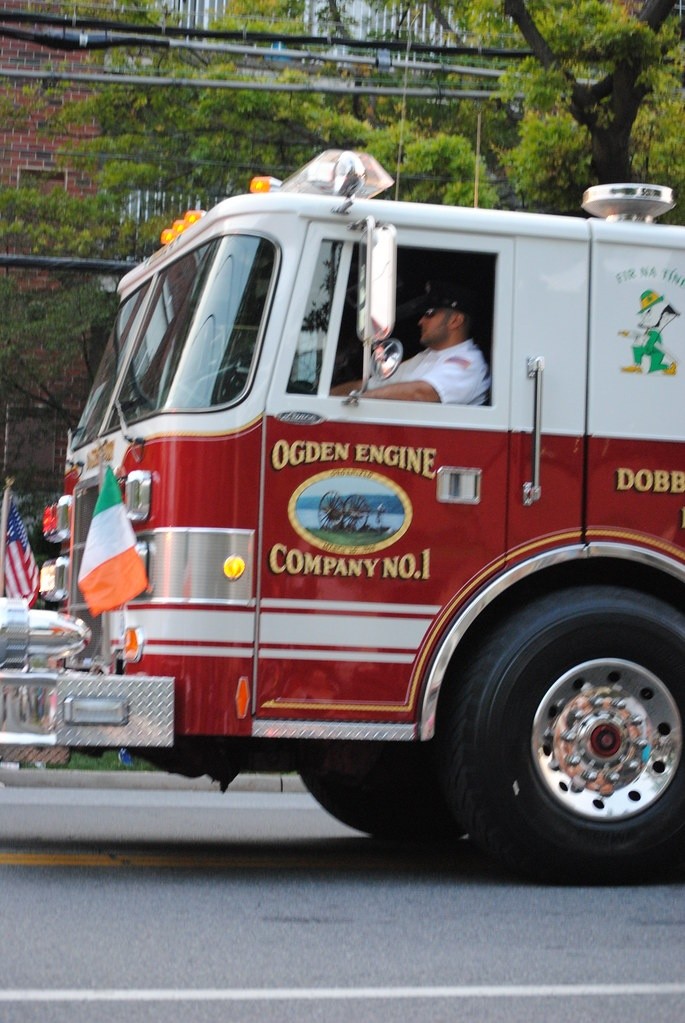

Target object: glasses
[421,308,441,318]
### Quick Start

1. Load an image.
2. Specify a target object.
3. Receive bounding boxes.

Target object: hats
[410,276,488,313]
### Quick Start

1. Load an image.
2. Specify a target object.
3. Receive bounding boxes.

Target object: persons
[118,749,133,765]
[330,281,490,404]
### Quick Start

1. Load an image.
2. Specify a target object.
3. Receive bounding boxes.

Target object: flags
[77,465,147,618]
[4,500,40,607]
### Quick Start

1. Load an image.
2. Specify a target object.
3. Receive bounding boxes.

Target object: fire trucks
[3,150,684,885]
